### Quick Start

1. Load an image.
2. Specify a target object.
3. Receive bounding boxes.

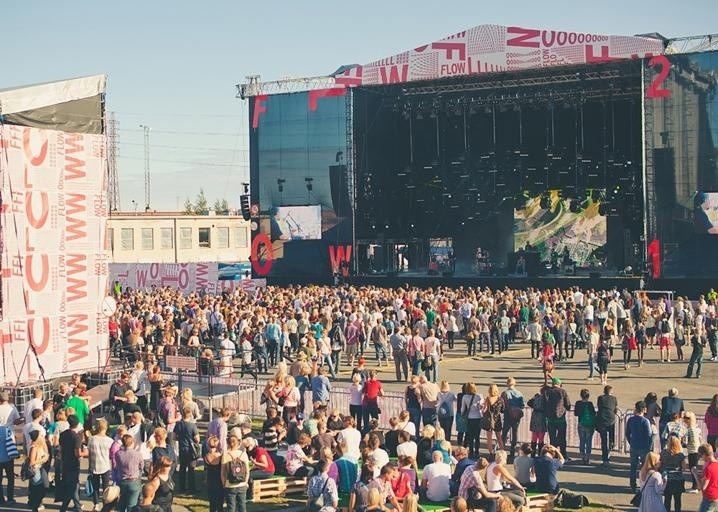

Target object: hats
[297,412,303,420]
[29,430,38,440]
[297,351,307,360]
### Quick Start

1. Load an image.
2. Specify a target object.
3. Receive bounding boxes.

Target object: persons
[269,206,297,241]
[692,190,718,238]
[0,280,717,512]
[446,247,483,272]
[517,242,569,275]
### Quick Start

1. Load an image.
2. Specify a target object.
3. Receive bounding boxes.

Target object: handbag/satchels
[415,349,433,370]
[85,475,93,496]
[630,492,642,507]
[544,359,553,370]
[278,397,286,406]
[458,415,467,424]
[310,494,324,510]
[557,490,584,508]
[189,438,200,459]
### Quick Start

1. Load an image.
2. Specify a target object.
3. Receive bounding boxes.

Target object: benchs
[89,425,625,511]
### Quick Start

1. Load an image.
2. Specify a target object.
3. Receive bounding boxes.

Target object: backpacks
[21,447,35,481]
[226,452,246,483]
[481,412,492,431]
[581,405,593,426]
[438,402,450,420]
